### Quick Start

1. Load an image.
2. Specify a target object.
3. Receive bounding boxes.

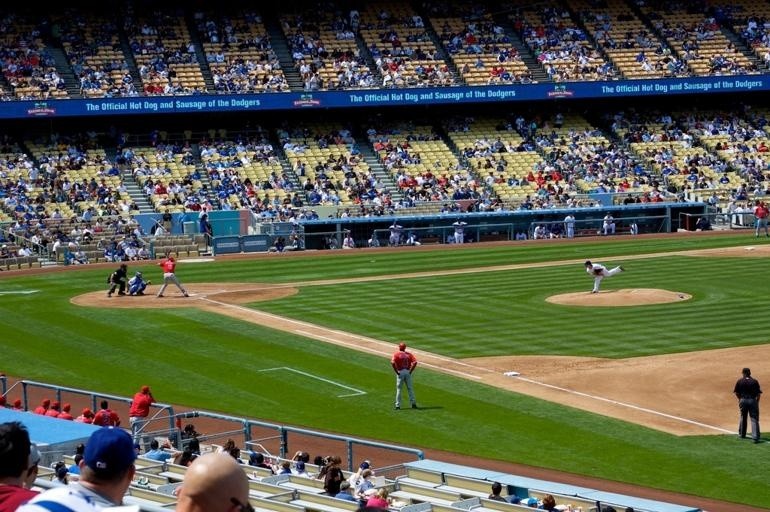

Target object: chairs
[3,3,769,269]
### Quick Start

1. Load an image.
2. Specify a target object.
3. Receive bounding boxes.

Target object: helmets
[400,342,407,350]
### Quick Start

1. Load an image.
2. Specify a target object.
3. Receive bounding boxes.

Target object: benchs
[32,450,630,512]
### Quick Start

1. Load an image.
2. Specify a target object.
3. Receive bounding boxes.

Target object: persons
[391,343,417,410]
[1,0,770,266]
[584,261,625,294]
[490,482,558,511]
[0,386,273,511]
[732,368,763,445]
[155,256,189,297]
[108,264,129,298]
[276,450,392,512]
[605,506,633,511]
[127,271,153,296]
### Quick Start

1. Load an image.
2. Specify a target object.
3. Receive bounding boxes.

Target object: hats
[141,386,149,392]
[14,400,21,405]
[0,397,6,404]
[57,467,69,475]
[82,408,91,415]
[42,399,70,411]
[363,469,375,477]
[296,461,304,471]
[83,426,138,476]
